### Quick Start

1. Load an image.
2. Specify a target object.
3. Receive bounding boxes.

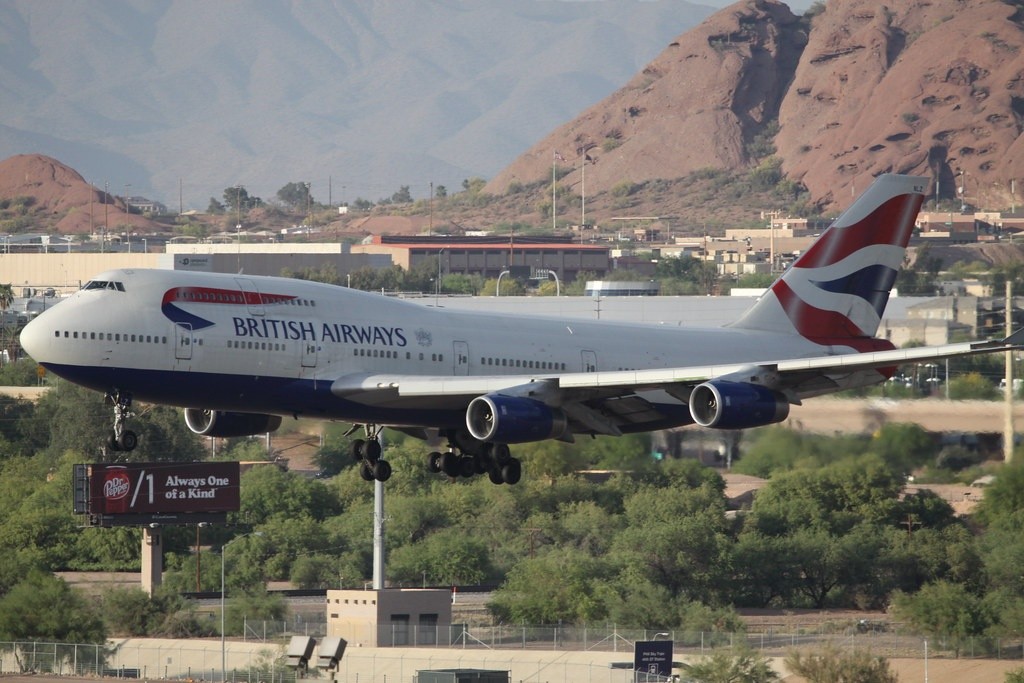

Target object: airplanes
[17,175,1023,488]
